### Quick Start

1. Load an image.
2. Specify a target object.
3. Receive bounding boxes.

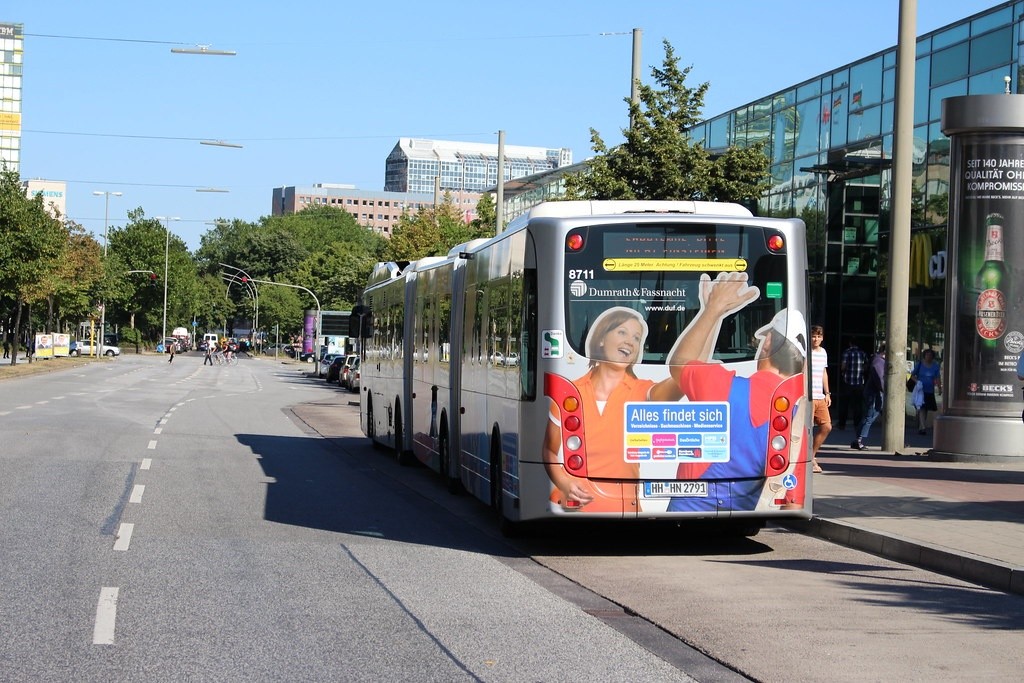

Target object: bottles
[976,214,1010,368]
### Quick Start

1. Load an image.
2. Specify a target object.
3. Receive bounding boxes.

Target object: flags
[817,105,831,123]
[853,90,862,104]
[833,94,841,108]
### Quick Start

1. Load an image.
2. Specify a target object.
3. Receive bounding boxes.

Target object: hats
[754,306,806,359]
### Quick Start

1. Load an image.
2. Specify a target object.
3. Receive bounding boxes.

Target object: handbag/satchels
[907,360,921,392]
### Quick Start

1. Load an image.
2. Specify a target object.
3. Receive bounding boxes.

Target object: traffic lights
[151,274,157,279]
[242,277,249,282]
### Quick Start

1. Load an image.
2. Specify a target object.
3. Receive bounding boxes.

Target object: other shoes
[851,440,862,449]
[812,460,822,473]
[858,443,869,451]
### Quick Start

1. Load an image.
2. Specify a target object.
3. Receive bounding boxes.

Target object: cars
[223,342,240,353]
[350,357,362,372]
[69,339,119,357]
[349,363,362,392]
[327,356,344,382]
[339,354,358,386]
[285,345,303,359]
[155,336,182,353]
[197,340,209,350]
[301,345,328,364]
[319,353,341,378]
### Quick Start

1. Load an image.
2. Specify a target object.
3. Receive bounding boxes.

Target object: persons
[664,270,807,514]
[54,335,69,347]
[168,339,176,365]
[1015,348,1024,423]
[808,325,833,473]
[203,343,213,366]
[835,332,871,430]
[541,273,749,515]
[82,330,101,346]
[850,341,887,450]
[910,349,942,435]
[221,341,229,358]
[69,339,78,356]
[227,341,238,359]
[212,342,223,364]
[31,340,35,354]
[38,336,52,349]
[3,339,10,359]
[225,339,237,364]
[25,336,30,357]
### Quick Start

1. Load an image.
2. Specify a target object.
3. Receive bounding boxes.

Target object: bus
[348,197,817,541]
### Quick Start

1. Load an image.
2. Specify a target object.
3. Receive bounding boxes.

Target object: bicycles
[204,350,239,366]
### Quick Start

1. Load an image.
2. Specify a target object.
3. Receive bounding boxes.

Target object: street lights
[92,190,124,359]
[155,215,180,354]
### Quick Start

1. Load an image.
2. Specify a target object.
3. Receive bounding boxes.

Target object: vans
[204,334,218,349]
[172,327,193,352]
[262,343,290,356]
